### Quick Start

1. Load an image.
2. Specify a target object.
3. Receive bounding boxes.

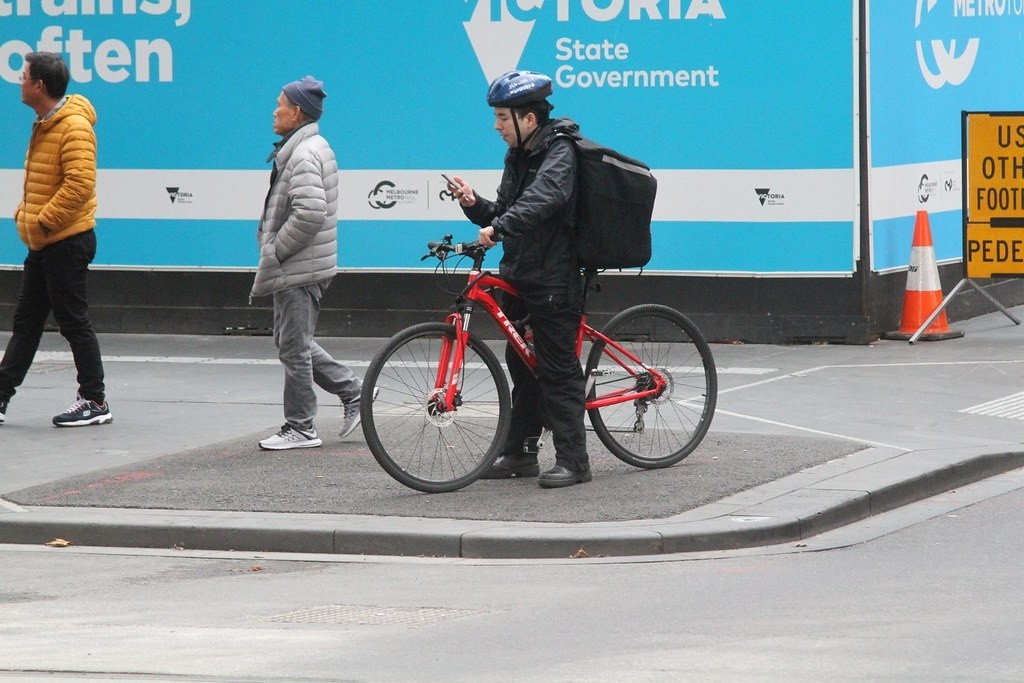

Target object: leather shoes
[478,454,541,480]
[539,466,592,488]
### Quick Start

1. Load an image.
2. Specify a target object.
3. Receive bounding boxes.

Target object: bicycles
[357,224,721,494]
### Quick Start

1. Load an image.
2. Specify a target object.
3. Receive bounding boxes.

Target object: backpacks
[540,131,658,269]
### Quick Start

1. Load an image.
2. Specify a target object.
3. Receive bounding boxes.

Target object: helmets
[487,69,555,108]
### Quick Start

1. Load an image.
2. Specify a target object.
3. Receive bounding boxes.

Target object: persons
[447,70,593,489]
[0,51,114,427]
[248,75,380,451]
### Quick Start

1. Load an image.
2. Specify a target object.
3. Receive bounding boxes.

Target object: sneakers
[340,385,380,439]
[53,392,114,426]
[0,400,7,421]
[259,424,323,450]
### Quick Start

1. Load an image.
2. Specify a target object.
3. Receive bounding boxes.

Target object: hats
[282,75,326,120]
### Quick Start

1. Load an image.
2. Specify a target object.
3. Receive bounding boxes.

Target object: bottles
[524,324,536,357]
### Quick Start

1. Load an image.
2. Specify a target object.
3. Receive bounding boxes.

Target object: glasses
[19,76,39,85]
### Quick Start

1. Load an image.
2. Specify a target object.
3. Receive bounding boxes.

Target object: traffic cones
[880,210,965,342]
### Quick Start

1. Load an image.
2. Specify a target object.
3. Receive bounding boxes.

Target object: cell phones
[441,172,473,202]
